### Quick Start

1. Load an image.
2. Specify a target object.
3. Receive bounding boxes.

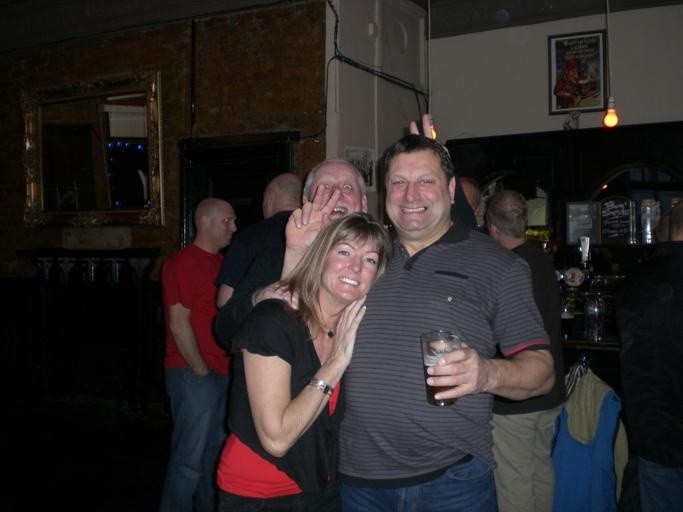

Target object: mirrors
[15,65,167,229]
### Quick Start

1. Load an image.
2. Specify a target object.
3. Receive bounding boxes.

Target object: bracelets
[309,377,335,396]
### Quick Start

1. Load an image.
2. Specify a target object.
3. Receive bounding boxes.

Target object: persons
[211,115,478,354]
[460,177,567,512]
[216,212,394,512]
[162,174,305,512]
[280,134,556,512]
[617,200,683,512]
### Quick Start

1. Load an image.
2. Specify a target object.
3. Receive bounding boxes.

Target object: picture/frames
[547,29,607,115]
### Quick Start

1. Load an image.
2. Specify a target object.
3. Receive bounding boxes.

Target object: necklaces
[321,324,337,338]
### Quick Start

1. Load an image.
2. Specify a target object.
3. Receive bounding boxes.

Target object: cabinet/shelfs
[444,120,683,367]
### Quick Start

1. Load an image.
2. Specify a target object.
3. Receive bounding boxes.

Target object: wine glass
[583,295,607,344]
[635,200,662,244]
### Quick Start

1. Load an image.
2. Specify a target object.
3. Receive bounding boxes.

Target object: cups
[420,331,461,405]
